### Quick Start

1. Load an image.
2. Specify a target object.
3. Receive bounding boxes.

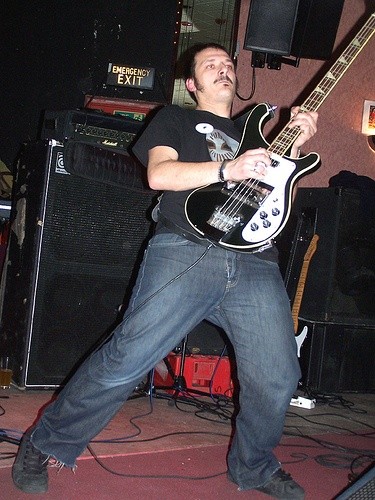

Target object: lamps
[367,135,375,153]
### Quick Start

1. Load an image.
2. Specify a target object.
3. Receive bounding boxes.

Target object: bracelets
[219,159,232,182]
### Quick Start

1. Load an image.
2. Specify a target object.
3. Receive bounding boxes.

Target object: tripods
[134,332,227,408]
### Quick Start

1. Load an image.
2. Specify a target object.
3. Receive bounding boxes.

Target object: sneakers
[12,426,48,494]
[254,467,305,500]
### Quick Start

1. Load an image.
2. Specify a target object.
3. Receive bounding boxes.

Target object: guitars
[180,10,375,255]
[290,233,320,391]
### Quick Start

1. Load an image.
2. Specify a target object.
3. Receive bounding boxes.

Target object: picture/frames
[361,99,375,136]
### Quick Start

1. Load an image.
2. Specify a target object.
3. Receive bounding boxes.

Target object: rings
[254,163,258,171]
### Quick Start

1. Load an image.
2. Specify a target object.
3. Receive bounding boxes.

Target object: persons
[11,43,318,500]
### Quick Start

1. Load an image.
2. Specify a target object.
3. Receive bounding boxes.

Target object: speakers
[243,0,314,57]
[273,188,375,324]
[175,319,236,356]
[0,137,165,392]
[332,465,375,500]
